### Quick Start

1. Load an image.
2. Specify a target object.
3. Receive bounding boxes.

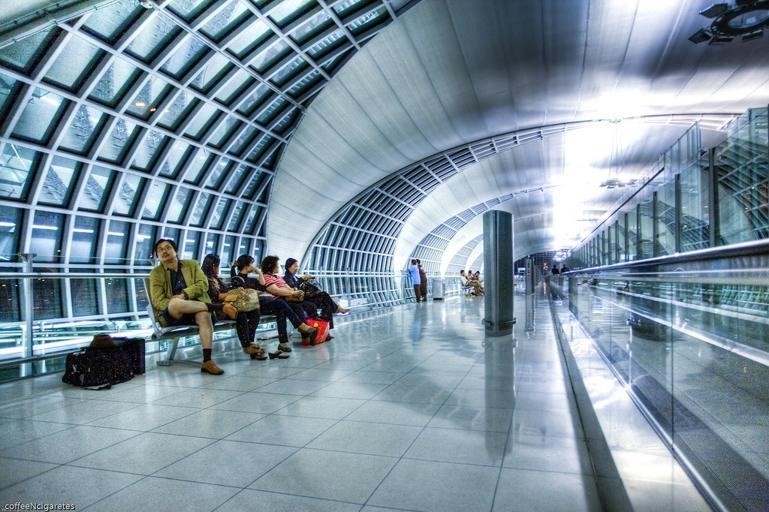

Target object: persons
[400,258,423,304]
[561,263,569,272]
[282,256,350,330]
[148,237,238,377]
[408,259,428,302]
[459,269,484,297]
[230,254,317,352]
[540,259,551,295]
[259,254,334,342]
[200,253,268,356]
[551,264,560,275]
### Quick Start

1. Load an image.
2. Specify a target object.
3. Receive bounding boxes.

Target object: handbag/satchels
[224,287,261,312]
[62,334,145,390]
[302,316,330,346]
[286,287,304,301]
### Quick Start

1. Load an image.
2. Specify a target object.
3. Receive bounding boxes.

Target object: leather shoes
[278,344,291,352]
[298,327,316,333]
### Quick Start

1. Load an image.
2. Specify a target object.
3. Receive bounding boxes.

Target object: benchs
[142,275,278,368]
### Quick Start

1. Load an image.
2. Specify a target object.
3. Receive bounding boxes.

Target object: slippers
[250,352,267,360]
[269,350,289,359]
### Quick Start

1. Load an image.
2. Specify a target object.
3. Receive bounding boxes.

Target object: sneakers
[336,306,350,314]
[201,360,224,375]
[223,303,238,319]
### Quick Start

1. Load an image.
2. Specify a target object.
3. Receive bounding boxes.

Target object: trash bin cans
[432,276,442,300]
